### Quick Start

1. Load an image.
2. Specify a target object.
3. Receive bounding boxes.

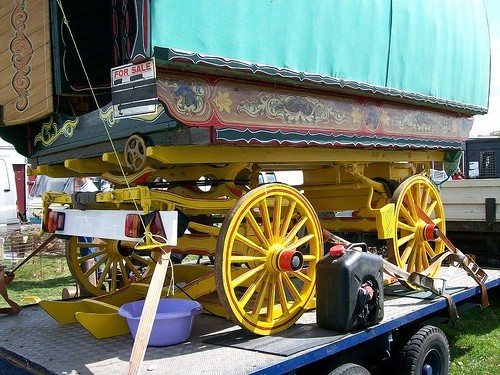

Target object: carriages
[0,0,490,338]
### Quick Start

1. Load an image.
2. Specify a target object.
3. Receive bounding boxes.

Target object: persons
[73,177,104,281]
[452,167,465,180]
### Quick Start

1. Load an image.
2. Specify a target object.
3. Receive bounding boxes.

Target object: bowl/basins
[117,298,204,346]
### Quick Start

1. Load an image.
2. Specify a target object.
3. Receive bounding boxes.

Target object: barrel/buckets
[315,242,384,332]
